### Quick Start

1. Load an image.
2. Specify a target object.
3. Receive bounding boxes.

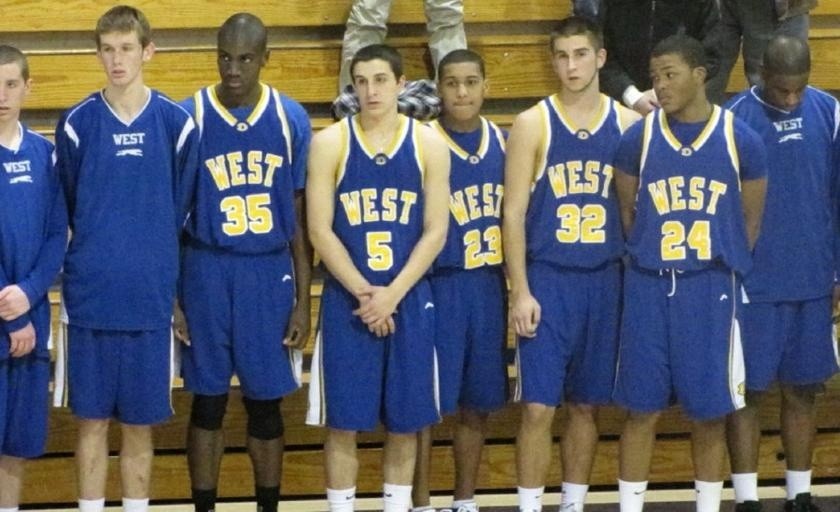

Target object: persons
[173,13,313,512]
[572,0,742,117]
[339,0,467,97]
[304,44,452,512]
[722,33,840,512]
[613,33,768,512]
[411,50,511,512]
[501,14,644,512]
[53,4,201,512]
[725,0,817,87]
[0,45,69,512]
